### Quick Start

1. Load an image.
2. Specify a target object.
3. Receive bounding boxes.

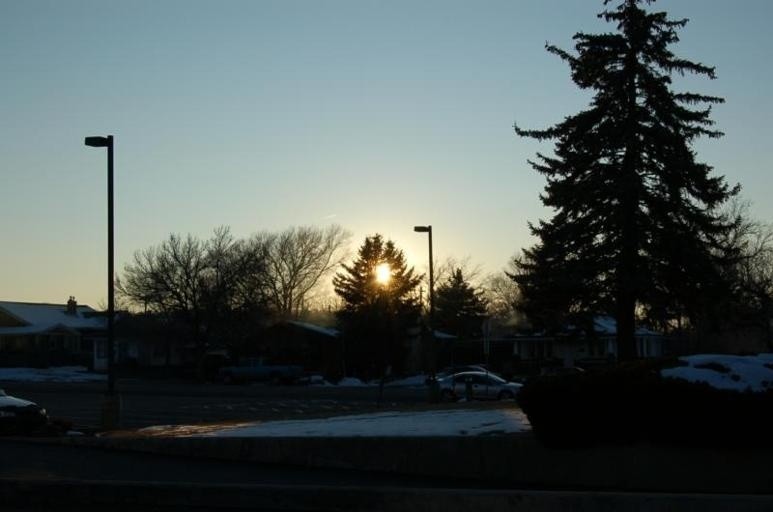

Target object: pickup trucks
[219,357,295,385]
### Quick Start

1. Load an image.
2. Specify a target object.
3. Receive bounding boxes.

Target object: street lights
[412,222,437,384]
[83,134,117,395]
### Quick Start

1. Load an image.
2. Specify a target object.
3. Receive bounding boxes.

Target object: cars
[431,363,524,405]
[0,387,48,437]
